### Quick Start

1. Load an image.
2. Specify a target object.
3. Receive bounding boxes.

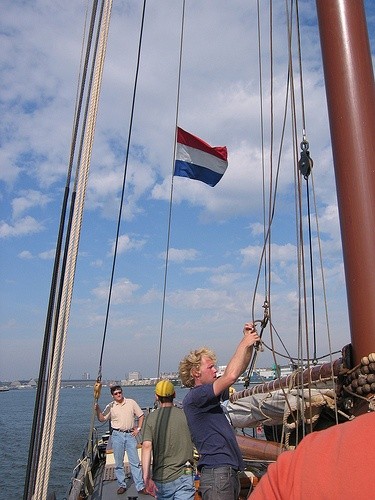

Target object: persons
[142,379,196,500]
[177,322,261,500]
[95,386,149,495]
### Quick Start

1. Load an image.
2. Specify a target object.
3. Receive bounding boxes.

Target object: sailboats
[23,0,375,499]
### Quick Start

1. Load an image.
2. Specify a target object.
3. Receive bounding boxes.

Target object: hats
[154,380,174,396]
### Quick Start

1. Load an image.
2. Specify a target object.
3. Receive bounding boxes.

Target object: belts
[114,428,134,433]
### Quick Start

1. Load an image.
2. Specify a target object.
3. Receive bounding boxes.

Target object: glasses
[114,391,122,395]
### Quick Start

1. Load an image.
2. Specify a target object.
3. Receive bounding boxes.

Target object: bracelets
[137,428,141,431]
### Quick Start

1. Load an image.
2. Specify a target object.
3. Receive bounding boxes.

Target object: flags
[172,126,228,187]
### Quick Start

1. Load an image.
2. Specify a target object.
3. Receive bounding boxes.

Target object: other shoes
[138,488,149,494]
[117,488,126,494]
